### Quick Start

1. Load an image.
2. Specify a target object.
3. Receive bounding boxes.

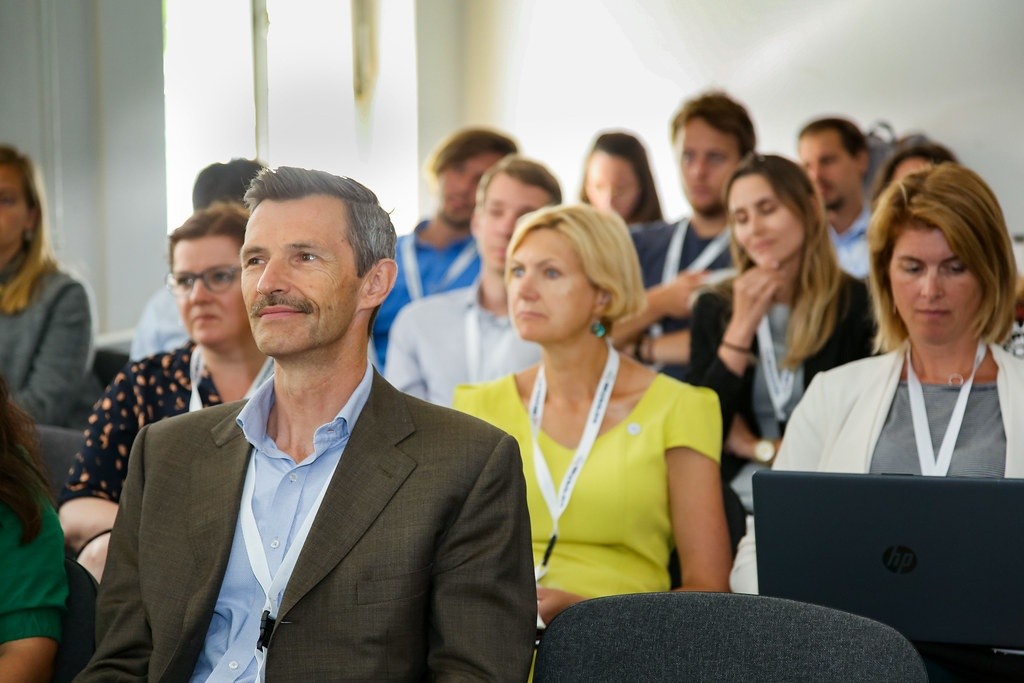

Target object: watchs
[755,436,776,466]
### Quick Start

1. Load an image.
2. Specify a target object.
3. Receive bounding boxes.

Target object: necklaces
[905,336,986,477]
[189,345,205,413]
[921,366,971,388]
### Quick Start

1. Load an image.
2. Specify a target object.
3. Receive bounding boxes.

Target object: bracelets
[648,336,655,364]
[635,337,644,361]
[721,340,752,354]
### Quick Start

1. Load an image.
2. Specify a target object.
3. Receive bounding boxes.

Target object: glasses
[164,264,242,296]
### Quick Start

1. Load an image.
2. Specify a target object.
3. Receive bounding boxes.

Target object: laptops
[751,470,1024,648]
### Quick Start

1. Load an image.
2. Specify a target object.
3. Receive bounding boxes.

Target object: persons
[0,373,67,683]
[69,164,538,683]
[0,90,1024,683]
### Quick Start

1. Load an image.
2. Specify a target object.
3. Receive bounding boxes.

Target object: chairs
[26,418,84,497]
[533,591,931,683]
